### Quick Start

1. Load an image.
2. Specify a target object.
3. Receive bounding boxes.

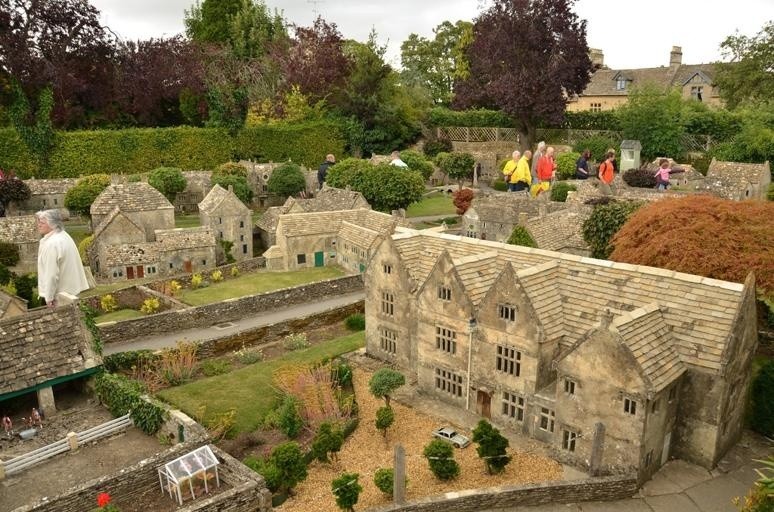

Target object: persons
[575,147,591,179]
[500,150,518,191]
[538,146,555,185]
[318,155,335,190]
[607,148,617,176]
[35,209,91,308]
[2,406,42,441]
[529,140,544,183]
[653,158,686,190]
[387,150,411,220]
[599,152,621,196]
[515,150,534,193]
[654,162,673,190]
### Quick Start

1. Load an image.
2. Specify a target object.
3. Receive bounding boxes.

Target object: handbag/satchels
[596,160,607,180]
[504,175,511,182]
[575,171,588,179]
